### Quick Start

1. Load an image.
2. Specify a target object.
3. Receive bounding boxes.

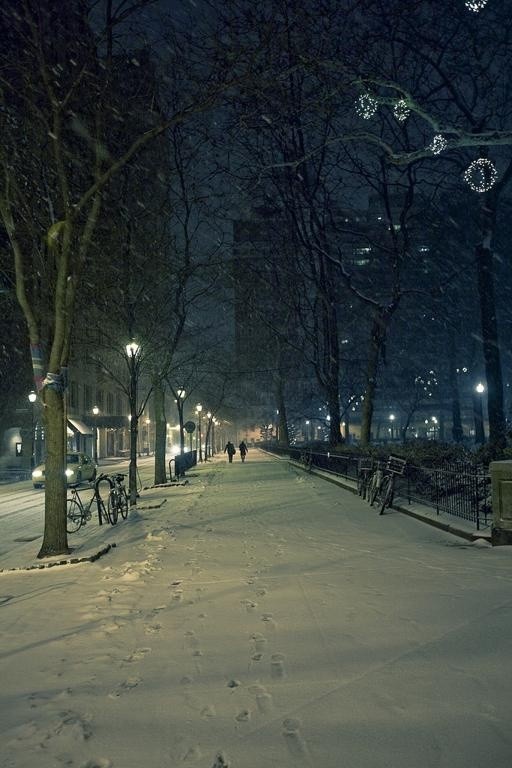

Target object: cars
[31,450,98,490]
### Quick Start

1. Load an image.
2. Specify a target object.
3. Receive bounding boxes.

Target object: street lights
[178,387,222,475]
[124,337,143,508]
[388,414,395,441]
[306,419,310,441]
[91,405,99,465]
[476,380,486,449]
[28,389,39,470]
[145,418,152,456]
[423,415,437,440]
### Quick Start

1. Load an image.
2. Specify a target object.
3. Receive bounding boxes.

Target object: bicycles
[67,470,110,534]
[106,471,129,529]
[350,450,406,515]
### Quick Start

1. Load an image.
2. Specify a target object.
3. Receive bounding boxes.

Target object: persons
[238,440,248,463]
[223,440,235,463]
